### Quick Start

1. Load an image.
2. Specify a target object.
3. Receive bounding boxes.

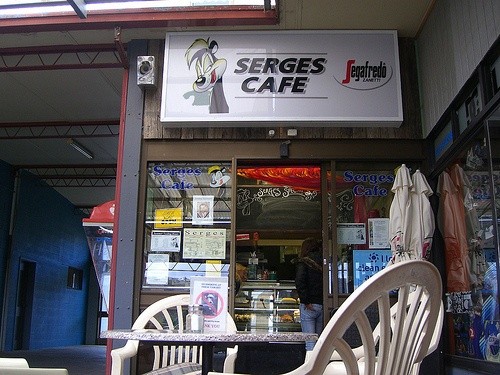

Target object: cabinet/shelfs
[234,280,302,332]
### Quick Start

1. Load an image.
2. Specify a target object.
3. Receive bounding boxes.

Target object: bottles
[186,304,204,334]
[263,271,269,280]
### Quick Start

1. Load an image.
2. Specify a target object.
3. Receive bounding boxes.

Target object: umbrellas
[384,163,436,316]
[438,165,487,315]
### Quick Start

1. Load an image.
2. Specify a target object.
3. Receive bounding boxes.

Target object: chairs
[281,257,445,375]
[110,295,239,375]
[0,358,70,375]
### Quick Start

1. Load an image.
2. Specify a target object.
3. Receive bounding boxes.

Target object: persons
[295,238,323,350]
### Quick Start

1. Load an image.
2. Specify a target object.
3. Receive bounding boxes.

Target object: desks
[100,329,318,375]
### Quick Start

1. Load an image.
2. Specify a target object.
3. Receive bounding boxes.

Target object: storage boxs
[256,252,265,260]
[237,251,251,260]
[267,271,278,280]
[246,264,258,280]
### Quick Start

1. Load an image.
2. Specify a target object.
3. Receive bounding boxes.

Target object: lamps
[66,138,95,161]
